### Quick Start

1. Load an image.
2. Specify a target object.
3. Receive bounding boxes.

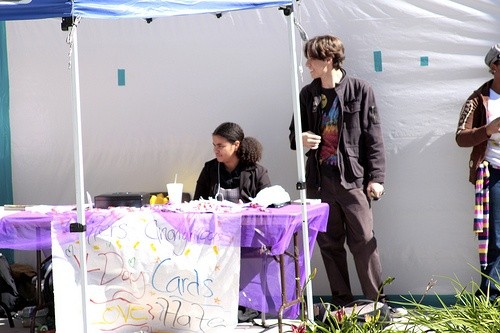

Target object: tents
[0,0,315,333]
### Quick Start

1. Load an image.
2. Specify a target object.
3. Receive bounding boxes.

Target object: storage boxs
[94,191,149,208]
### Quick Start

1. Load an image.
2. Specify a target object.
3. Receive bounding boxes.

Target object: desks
[0,202,329,333]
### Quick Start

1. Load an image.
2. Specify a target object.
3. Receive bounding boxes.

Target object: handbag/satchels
[9,270,38,311]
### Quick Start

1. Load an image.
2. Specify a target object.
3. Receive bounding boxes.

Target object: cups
[167,183,183,204]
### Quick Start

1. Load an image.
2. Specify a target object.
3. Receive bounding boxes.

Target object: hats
[485,44,500,67]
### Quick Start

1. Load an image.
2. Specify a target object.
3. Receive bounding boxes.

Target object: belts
[319,160,338,168]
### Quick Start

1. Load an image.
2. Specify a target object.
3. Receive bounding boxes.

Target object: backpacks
[29,255,54,318]
[0,254,20,328]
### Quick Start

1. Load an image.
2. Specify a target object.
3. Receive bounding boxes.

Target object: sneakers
[239,308,259,321]
[375,304,391,326]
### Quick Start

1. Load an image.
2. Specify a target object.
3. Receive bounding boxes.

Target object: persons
[455,44,500,304]
[193,122,271,203]
[289,35,390,329]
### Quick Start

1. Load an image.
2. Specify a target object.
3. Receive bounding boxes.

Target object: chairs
[150,192,190,203]
